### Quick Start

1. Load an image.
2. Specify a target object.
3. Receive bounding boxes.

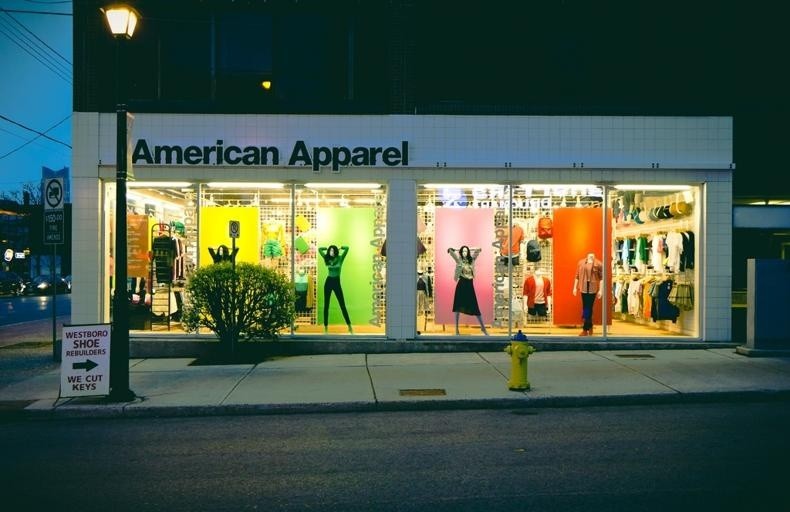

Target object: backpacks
[526,239,542,262]
[537,216,553,239]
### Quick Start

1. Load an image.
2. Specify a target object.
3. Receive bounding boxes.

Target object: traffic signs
[59,323,112,398]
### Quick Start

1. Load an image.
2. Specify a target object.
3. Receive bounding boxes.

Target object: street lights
[100,5,144,402]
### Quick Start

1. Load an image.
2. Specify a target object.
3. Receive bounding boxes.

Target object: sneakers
[579,325,593,336]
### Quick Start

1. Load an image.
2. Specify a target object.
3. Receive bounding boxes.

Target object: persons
[572,252,605,337]
[208,245,240,263]
[318,244,352,336]
[447,245,490,337]
[522,269,552,321]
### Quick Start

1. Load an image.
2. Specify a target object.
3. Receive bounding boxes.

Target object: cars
[0,271,71,295]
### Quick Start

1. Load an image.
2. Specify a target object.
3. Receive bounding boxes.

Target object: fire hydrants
[504,330,536,389]
